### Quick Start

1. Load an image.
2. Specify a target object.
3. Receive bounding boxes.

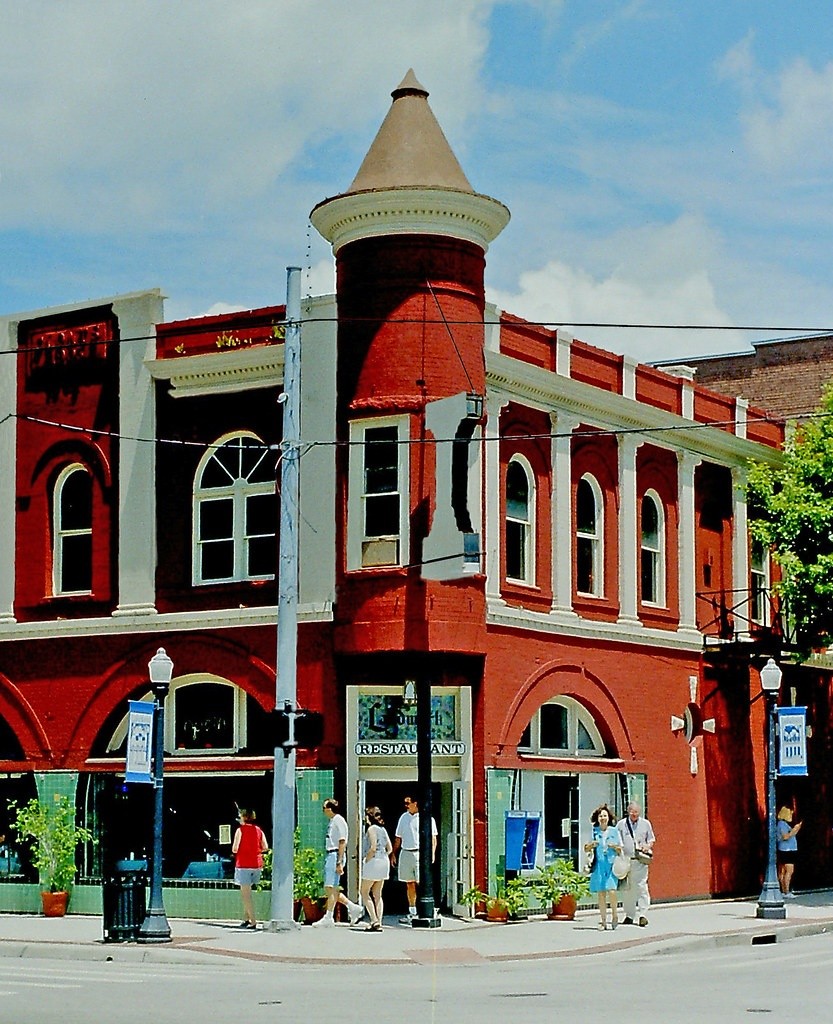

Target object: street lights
[137,647,172,944]
[755,658,786,918]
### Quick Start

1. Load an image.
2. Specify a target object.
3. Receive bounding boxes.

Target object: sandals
[363,922,384,932]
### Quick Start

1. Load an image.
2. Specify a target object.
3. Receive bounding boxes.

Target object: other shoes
[611,915,619,929]
[399,914,420,925]
[598,922,608,930]
[623,916,633,924]
[638,916,649,928]
[782,892,799,899]
[239,921,257,932]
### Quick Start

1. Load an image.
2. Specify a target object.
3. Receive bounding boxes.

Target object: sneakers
[348,904,364,926]
[312,915,334,928]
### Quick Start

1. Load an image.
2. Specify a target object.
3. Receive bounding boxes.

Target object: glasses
[323,806,330,811]
[403,801,414,807]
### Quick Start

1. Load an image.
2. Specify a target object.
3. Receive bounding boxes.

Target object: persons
[777,806,802,899]
[584,806,625,930]
[615,800,656,927]
[391,793,438,923]
[360,806,393,931]
[312,798,364,929]
[232,809,268,929]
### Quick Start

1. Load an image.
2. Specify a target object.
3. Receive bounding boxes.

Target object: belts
[327,848,341,854]
[406,849,419,852]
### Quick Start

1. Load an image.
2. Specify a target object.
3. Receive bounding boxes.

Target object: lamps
[403,679,416,706]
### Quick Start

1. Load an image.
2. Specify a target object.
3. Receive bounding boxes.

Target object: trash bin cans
[102,868,149,943]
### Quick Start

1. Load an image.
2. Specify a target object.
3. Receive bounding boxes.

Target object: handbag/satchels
[584,849,596,874]
[634,847,652,865]
[612,850,631,879]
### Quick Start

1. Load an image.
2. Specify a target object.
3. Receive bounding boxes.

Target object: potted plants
[462,874,531,924]
[257,826,345,925]
[6,793,92,913]
[533,858,594,921]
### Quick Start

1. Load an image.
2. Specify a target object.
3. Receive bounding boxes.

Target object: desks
[116,860,148,875]
[182,862,224,880]
